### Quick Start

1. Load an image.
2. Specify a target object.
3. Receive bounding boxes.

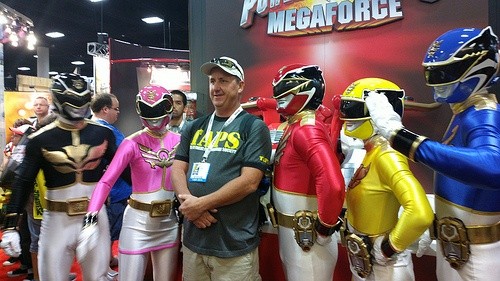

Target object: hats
[8,124,37,135]
[200,56,245,82]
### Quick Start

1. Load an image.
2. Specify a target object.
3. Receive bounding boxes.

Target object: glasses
[209,58,243,79]
[108,105,120,112]
[34,103,48,107]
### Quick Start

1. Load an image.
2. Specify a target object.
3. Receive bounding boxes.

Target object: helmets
[338,76,405,139]
[271,63,324,116]
[135,85,174,129]
[51,73,91,121]
[421,27,500,105]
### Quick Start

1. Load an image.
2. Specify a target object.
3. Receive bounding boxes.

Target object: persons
[183,90,201,122]
[271,62,347,281]
[364,25,499,281]
[76,82,186,281]
[2,124,34,268]
[2,119,37,276]
[170,54,274,281]
[84,92,139,281]
[0,72,142,281]
[339,75,434,281]
[24,112,79,279]
[32,96,53,122]
[161,87,193,141]
